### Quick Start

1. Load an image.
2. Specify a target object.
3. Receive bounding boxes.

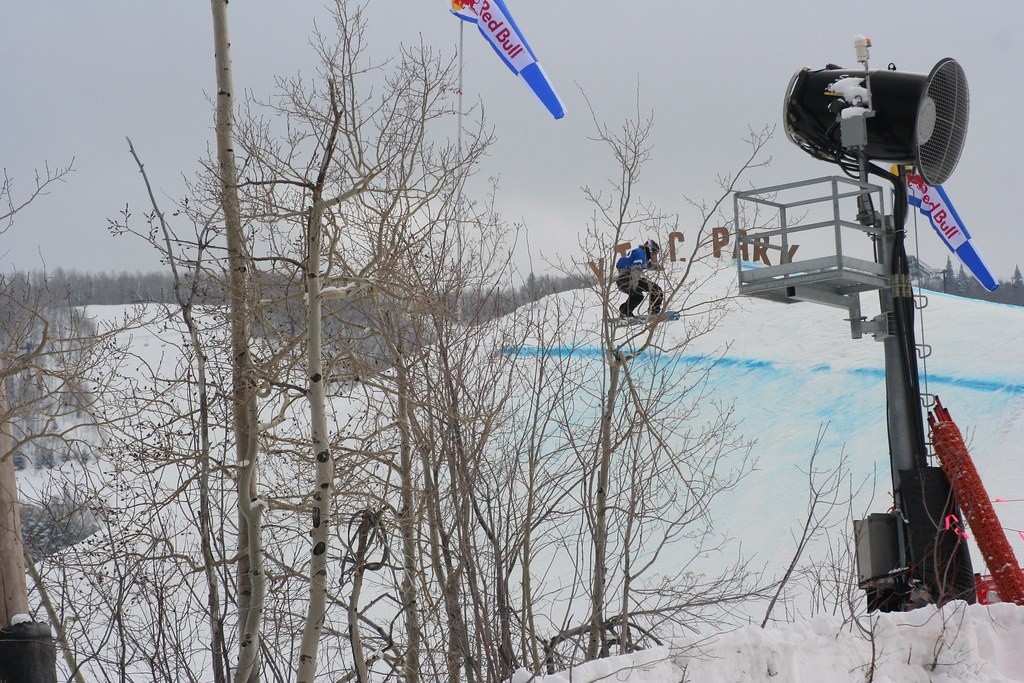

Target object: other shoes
[620,307,634,317]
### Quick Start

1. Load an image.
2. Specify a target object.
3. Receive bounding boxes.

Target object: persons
[615,239,664,318]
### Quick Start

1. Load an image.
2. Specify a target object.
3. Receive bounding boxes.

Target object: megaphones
[783,57,970,186]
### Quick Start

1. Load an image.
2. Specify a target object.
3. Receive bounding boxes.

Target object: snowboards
[601,310,683,325]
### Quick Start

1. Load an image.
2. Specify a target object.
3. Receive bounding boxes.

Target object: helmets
[645,239,659,259]
